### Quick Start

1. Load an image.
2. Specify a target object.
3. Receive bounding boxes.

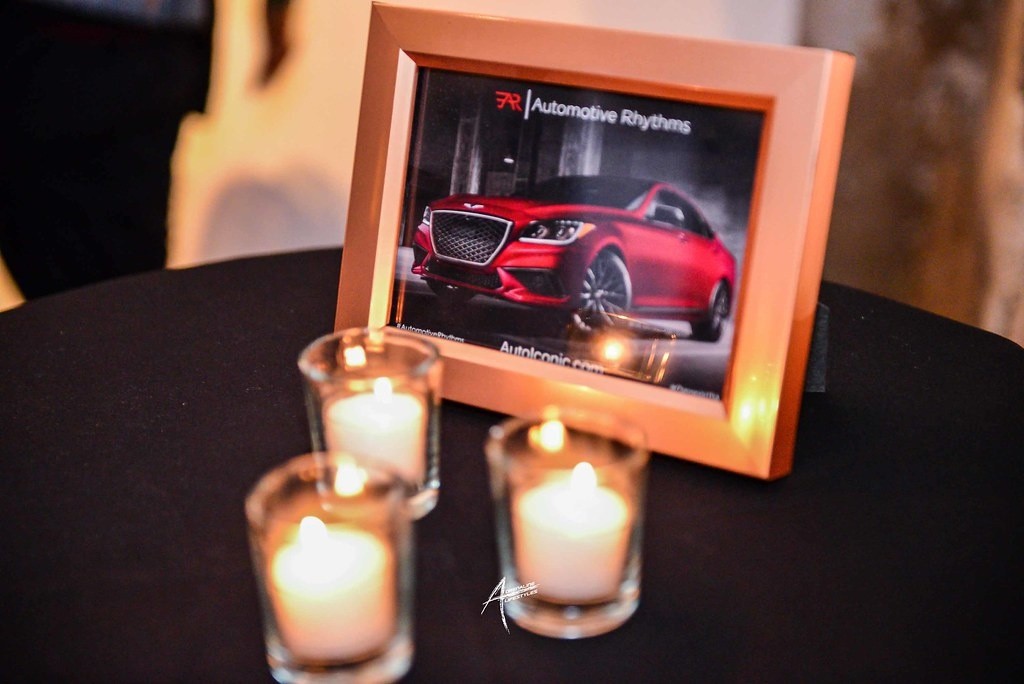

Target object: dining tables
[0,243,1024,684]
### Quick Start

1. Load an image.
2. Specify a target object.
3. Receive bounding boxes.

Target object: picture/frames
[333,1,858,480]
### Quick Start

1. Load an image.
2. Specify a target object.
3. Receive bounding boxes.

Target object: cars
[408,171,739,342]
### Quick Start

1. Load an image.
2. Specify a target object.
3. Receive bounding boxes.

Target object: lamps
[296,326,444,524]
[484,404,651,641]
[242,451,419,684]
